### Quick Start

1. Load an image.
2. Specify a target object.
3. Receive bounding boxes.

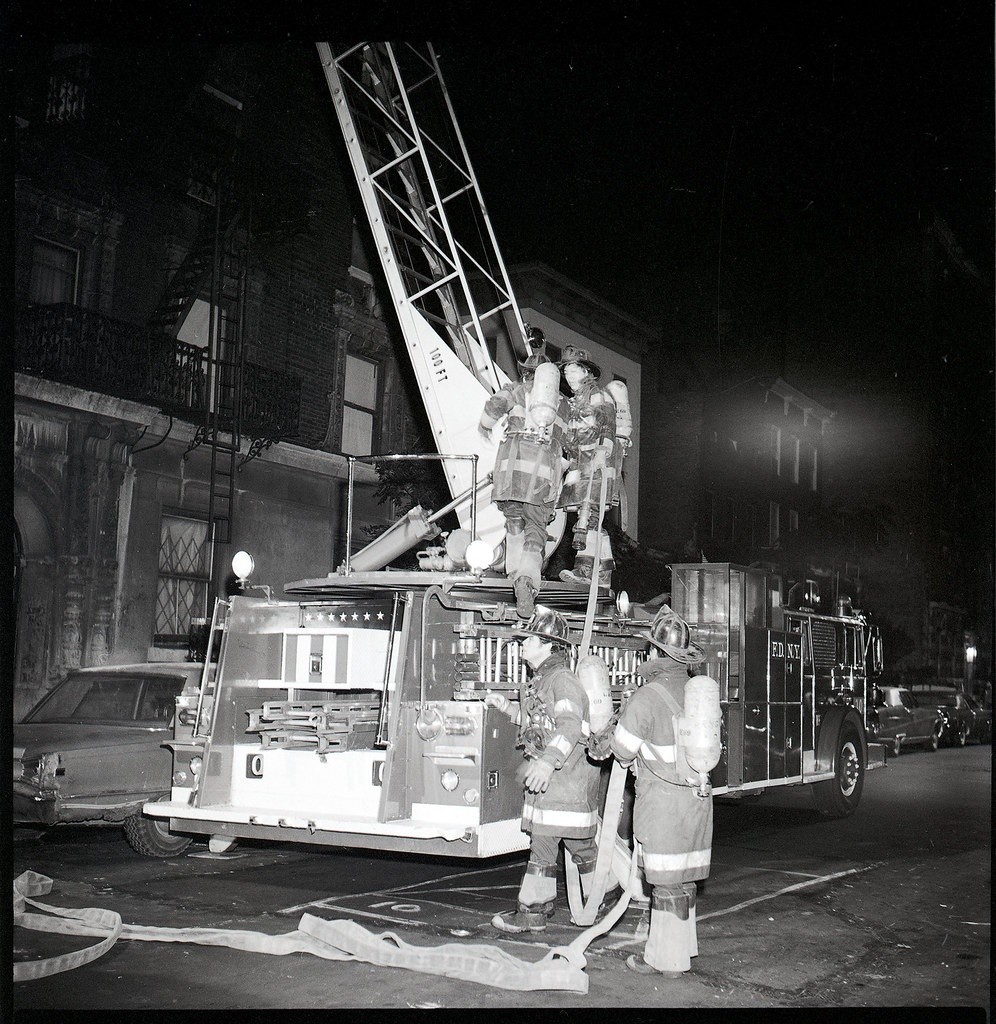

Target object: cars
[875,686,945,757]
[911,689,993,747]
[9,662,226,857]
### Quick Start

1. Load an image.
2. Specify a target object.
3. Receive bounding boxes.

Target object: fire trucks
[140,13,885,865]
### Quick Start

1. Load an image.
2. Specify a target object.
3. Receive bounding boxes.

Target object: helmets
[558,346,603,382]
[638,604,706,664]
[521,609,573,645]
[517,353,550,369]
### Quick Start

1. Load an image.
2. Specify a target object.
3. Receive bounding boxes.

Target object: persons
[612,615,714,974]
[559,348,620,589]
[484,612,602,933]
[478,353,575,620]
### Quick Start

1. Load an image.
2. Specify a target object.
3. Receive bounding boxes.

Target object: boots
[571,858,619,925]
[596,535,616,587]
[513,542,543,617]
[504,521,525,579]
[491,861,558,932]
[560,530,597,584]
[683,883,699,958]
[625,890,691,979]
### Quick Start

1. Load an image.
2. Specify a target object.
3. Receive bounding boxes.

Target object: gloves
[524,754,557,793]
[484,692,516,716]
